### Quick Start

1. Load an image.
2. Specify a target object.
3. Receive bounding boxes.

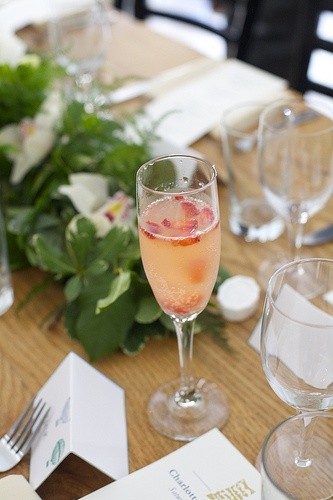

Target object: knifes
[303,223,333,246]
[103,58,204,107]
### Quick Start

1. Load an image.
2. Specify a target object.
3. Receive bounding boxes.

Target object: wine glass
[260,258,333,500]
[47,0,111,120]
[256,99,333,302]
[136,155,230,441]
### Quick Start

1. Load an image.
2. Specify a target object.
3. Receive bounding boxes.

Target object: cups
[0,181,15,317]
[221,103,295,243]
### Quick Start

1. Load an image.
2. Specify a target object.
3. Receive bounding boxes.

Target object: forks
[0,397,51,472]
[225,108,320,154]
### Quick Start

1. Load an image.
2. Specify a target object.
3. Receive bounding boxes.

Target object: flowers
[0,48,232,365]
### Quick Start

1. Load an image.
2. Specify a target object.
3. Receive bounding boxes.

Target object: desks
[0,15,333,500]
